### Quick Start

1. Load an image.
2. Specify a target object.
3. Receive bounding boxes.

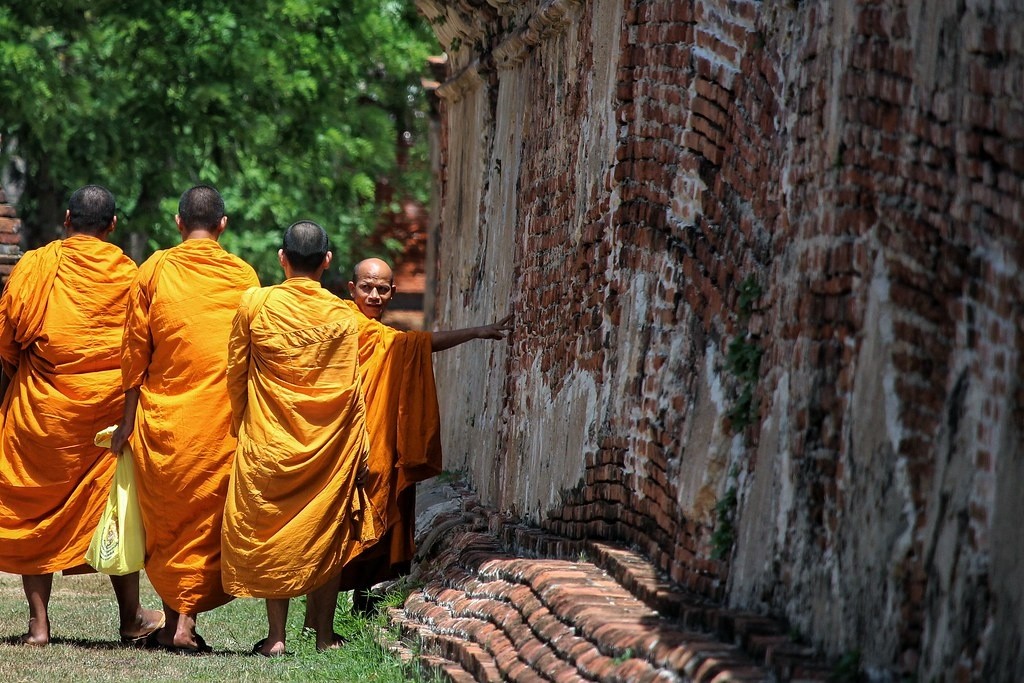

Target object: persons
[301,258,514,640]
[0,185,166,646]
[220,221,378,656]
[111,186,261,651]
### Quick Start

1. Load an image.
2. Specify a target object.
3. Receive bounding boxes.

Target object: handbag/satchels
[84,424,145,578]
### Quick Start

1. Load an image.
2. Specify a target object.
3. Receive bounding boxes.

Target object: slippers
[135,628,173,650]
[252,637,287,658]
[316,633,350,654]
[171,632,212,653]
[116,608,166,643]
[11,633,51,651]
